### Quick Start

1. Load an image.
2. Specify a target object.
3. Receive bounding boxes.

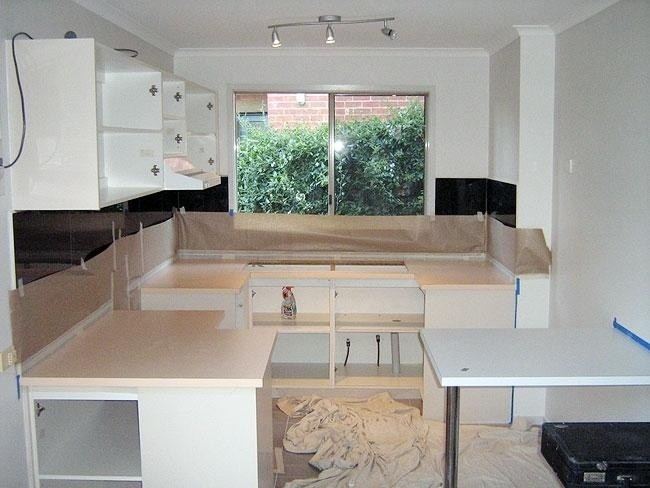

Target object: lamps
[267,16,398,49]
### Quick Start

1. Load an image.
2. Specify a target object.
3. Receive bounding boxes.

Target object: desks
[421,330,650,488]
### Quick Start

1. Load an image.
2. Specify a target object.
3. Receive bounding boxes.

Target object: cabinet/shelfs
[6,39,221,211]
[239,278,424,412]
[24,393,143,488]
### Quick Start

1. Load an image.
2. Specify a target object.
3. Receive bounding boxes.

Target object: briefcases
[542,422,650,488]
